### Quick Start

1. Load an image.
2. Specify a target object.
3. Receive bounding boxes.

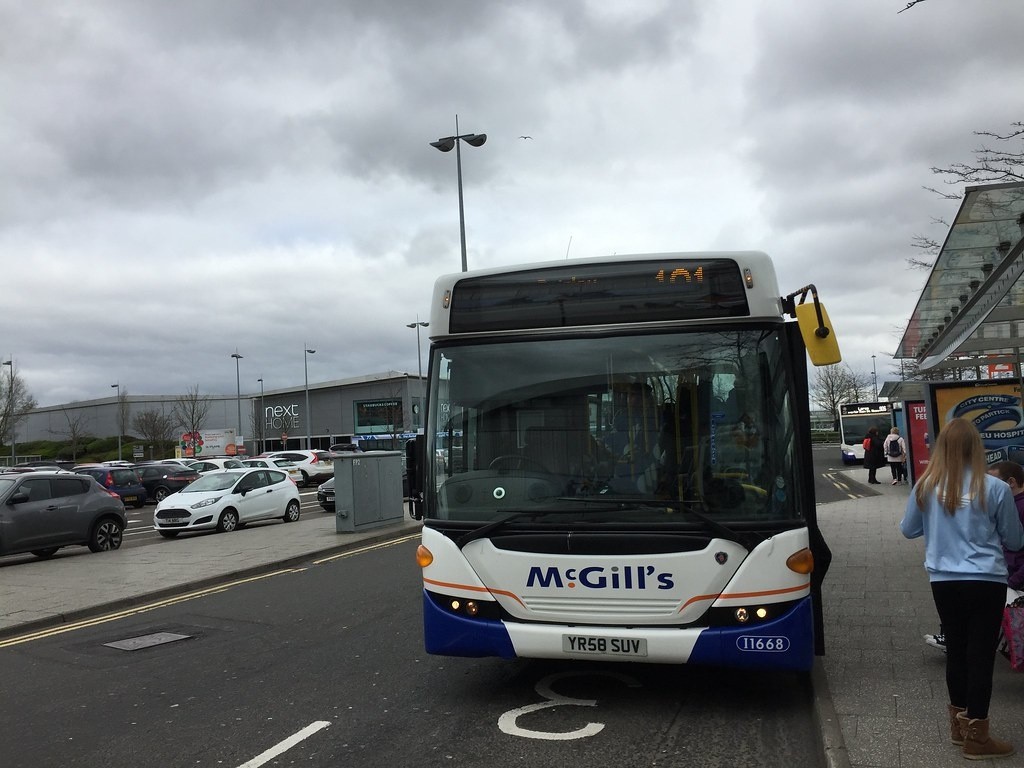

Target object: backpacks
[862,438,872,451]
[888,436,903,457]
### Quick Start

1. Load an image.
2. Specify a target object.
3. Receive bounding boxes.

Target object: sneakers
[922,633,947,649]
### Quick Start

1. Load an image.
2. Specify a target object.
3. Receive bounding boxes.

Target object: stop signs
[281,433,288,441]
[238,447,246,454]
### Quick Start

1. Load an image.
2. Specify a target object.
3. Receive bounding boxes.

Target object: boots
[948,705,1016,760]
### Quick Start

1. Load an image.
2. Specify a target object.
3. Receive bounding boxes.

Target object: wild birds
[518,136,533,140]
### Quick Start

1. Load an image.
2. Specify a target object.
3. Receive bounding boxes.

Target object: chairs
[245,473,260,483]
[519,426,590,486]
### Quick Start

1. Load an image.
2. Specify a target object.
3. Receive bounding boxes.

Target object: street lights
[431,133,488,274]
[305,350,316,448]
[232,354,244,458]
[871,354,879,402]
[407,323,431,427]
[258,379,265,452]
[3,361,15,467]
[111,384,121,461]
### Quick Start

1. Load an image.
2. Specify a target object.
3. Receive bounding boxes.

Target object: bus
[406,250,842,668]
[833,402,896,465]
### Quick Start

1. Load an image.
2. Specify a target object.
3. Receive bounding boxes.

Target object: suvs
[0,472,128,557]
[258,449,334,487]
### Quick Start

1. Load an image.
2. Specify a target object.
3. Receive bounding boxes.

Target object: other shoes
[892,479,902,485]
[868,479,881,484]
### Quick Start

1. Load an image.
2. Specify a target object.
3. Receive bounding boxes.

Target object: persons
[922,461,1024,649]
[899,418,1024,760]
[884,427,909,485]
[864,427,886,484]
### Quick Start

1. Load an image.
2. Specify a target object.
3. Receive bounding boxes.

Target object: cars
[131,465,202,504]
[153,467,301,537]
[77,468,146,508]
[0,449,303,489]
[316,464,407,513]
[329,444,463,472]
